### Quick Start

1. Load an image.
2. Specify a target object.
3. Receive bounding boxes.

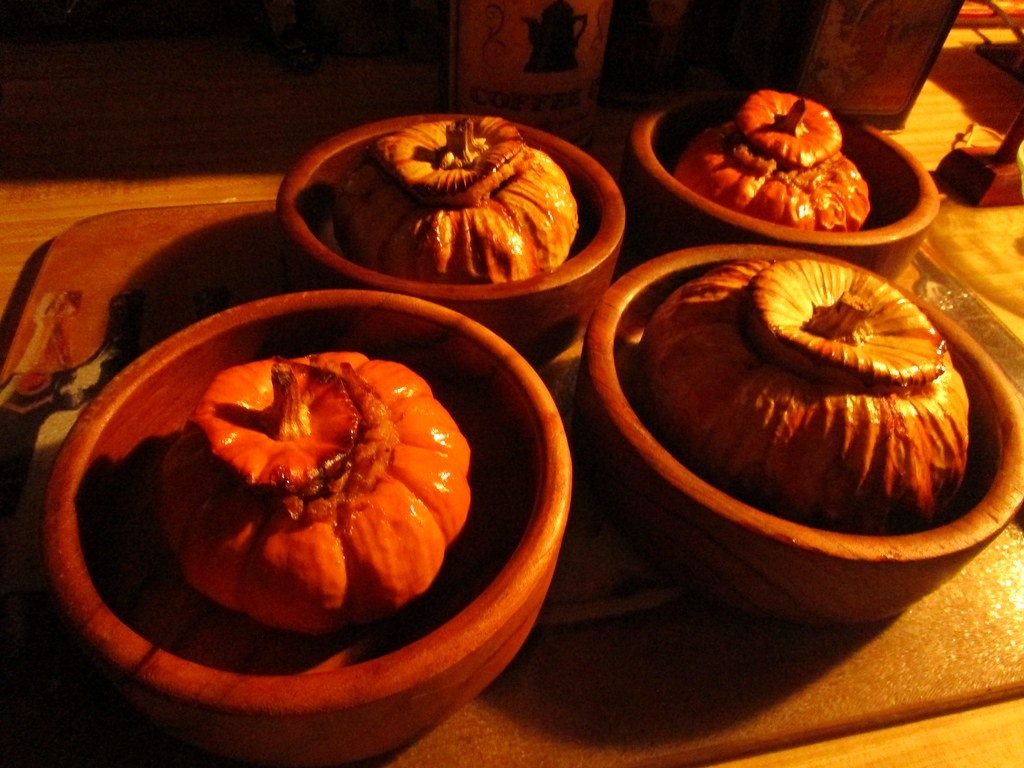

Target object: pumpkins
[159,89,971,638]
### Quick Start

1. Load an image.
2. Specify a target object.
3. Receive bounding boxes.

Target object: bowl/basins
[626,88,940,301]
[41,286,572,766]
[576,244,1023,626]
[276,114,626,376]
